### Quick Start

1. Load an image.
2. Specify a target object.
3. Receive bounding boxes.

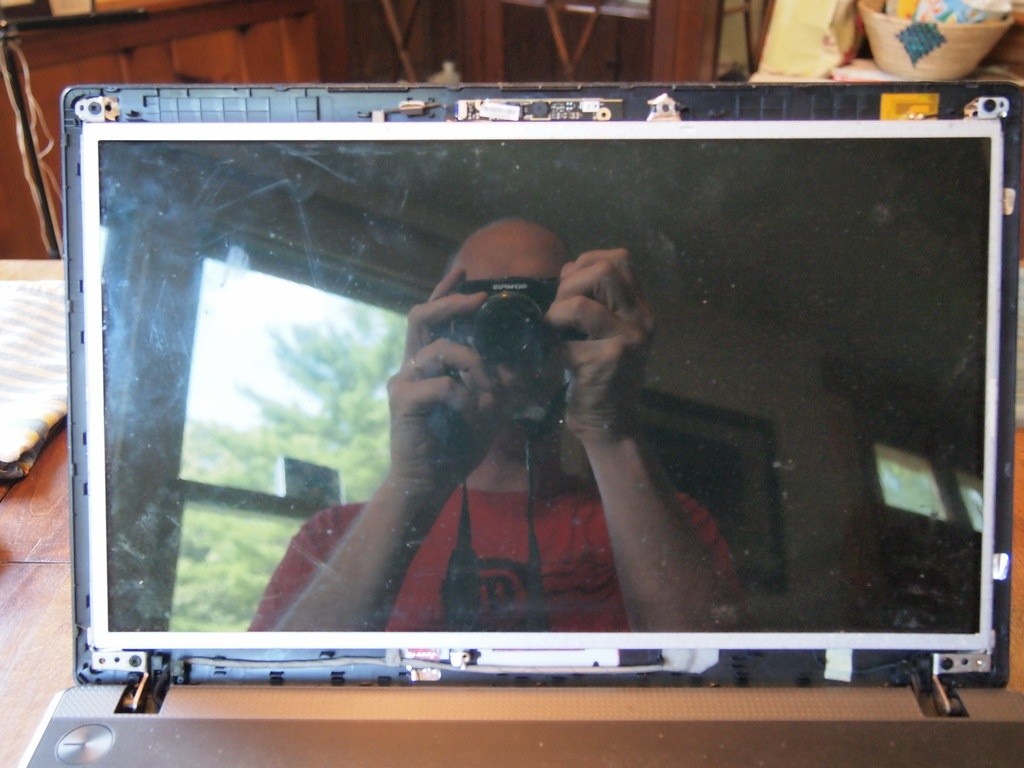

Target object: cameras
[448,274,586,367]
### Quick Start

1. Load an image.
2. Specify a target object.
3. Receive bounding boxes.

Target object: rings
[410,358,421,371]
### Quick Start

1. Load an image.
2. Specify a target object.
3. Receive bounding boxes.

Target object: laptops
[17,83,1024,768]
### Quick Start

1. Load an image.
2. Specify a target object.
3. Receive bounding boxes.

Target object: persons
[248,215,737,631]
